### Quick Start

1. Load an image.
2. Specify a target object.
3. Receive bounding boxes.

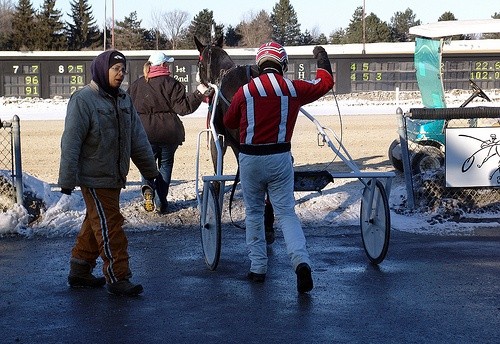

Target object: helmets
[256,42,288,74]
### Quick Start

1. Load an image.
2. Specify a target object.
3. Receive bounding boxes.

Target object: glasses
[110,65,126,74]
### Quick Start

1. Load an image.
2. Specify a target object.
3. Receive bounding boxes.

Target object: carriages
[193,34,397,271]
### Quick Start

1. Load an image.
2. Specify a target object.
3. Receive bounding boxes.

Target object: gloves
[312,46,329,61]
[143,169,163,185]
[60,187,75,195]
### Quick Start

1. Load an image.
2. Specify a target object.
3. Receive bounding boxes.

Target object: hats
[148,53,175,66]
[108,52,125,71]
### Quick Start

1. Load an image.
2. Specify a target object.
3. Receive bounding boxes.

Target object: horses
[192,32,261,204]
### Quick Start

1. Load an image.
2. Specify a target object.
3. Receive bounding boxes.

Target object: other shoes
[156,202,169,213]
[143,188,154,211]
[247,271,266,283]
[107,279,144,294]
[68,271,106,288]
[295,263,325,288]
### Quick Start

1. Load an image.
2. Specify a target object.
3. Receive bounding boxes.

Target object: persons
[58,51,164,296]
[124,51,208,218]
[223,42,334,293]
